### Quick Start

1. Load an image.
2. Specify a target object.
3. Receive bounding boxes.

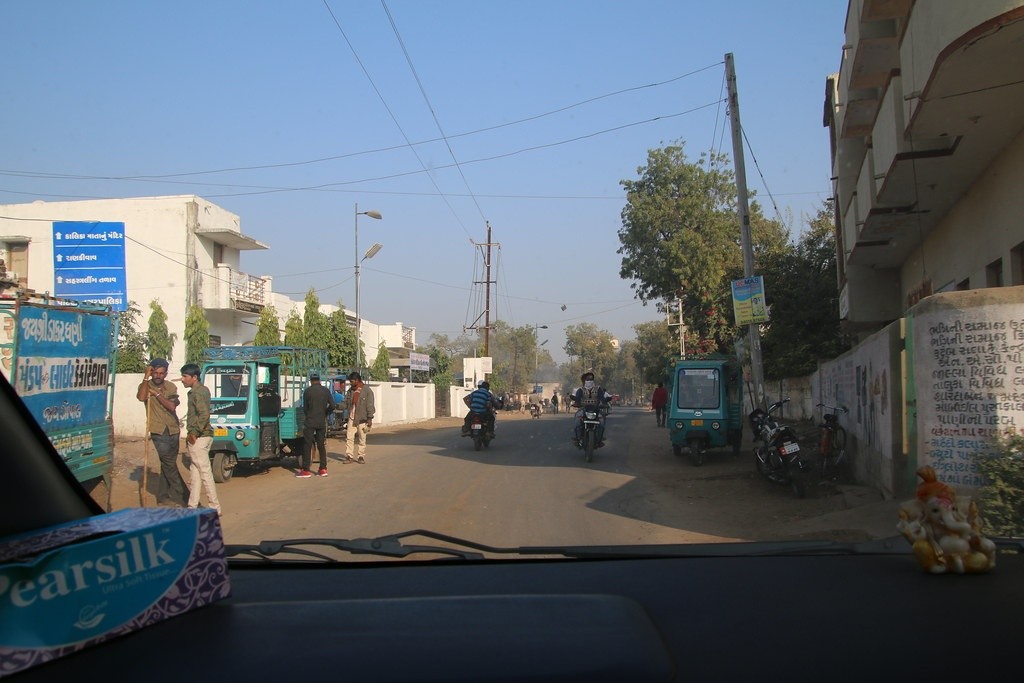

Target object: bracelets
[144,379,147,381]
[156,394,160,397]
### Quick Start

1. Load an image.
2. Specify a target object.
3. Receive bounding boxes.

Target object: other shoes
[486,432,495,438]
[571,436,577,440]
[572,440,578,446]
[603,437,606,440]
[358,457,365,464]
[599,442,604,447]
[461,432,471,437]
[343,457,353,464]
[657,424,661,427]
[662,424,665,427]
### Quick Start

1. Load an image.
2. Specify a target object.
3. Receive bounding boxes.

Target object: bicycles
[816,402,849,480]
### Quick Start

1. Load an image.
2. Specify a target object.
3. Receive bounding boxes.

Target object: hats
[151,358,169,368]
[309,374,320,380]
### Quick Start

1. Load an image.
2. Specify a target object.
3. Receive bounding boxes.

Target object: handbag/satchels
[651,402,656,410]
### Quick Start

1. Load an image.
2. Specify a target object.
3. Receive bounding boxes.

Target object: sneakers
[296,469,311,476]
[316,468,328,476]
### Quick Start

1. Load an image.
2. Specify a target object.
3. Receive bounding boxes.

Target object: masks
[585,381,594,389]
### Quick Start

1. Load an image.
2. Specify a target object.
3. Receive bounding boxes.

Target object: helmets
[478,380,484,385]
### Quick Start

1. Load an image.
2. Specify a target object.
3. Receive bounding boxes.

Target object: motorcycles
[747,398,812,499]
[0,277,122,493]
[569,394,613,464]
[664,355,745,465]
[196,345,326,484]
[468,396,504,451]
[529,398,544,420]
[290,367,371,439]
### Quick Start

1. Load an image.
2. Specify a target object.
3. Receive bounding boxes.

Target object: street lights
[353,201,384,377]
[534,323,549,394]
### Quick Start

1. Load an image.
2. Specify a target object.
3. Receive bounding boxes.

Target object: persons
[461,380,502,439]
[564,393,571,413]
[181,364,221,514]
[295,374,334,477]
[529,390,543,413]
[552,392,558,415]
[571,373,614,447]
[652,383,668,428]
[138,358,190,505]
[343,372,376,464]
[603,389,611,398]
